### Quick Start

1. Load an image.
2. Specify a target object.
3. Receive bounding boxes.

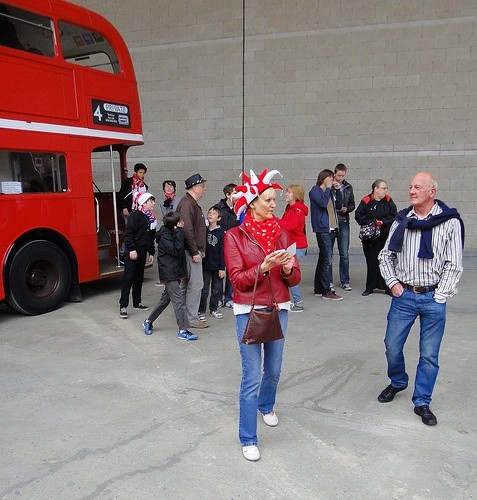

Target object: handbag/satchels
[358,221,381,241]
[241,262,284,345]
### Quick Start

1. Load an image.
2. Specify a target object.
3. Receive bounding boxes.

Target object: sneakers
[315,290,343,300]
[115,255,125,264]
[290,305,304,312]
[142,319,153,335]
[188,320,208,328]
[217,300,234,311]
[133,305,149,311]
[330,284,352,291]
[242,445,260,461]
[120,308,128,318]
[177,330,198,340]
[262,410,279,427]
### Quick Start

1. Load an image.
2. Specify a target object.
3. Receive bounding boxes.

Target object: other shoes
[154,282,165,287]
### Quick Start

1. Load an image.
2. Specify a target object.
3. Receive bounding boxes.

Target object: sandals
[210,311,223,319]
[198,313,206,321]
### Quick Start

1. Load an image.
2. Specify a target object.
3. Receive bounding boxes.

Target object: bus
[0,0,160,315]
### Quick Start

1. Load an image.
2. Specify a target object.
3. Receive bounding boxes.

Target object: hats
[231,168,284,220]
[138,193,153,206]
[184,174,207,190]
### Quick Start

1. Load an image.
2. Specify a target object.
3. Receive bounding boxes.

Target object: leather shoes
[414,406,437,425]
[362,288,392,297]
[378,381,407,403]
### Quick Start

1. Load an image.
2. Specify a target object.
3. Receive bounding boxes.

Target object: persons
[212,183,240,310]
[114,162,149,264]
[271,183,309,313]
[119,193,159,318]
[308,169,345,301]
[154,180,182,287]
[174,173,211,329]
[141,211,199,341]
[197,204,226,322]
[354,177,399,299]
[327,163,356,292]
[222,167,302,462]
[376,170,466,426]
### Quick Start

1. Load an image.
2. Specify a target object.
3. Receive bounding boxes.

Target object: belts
[397,279,439,294]
